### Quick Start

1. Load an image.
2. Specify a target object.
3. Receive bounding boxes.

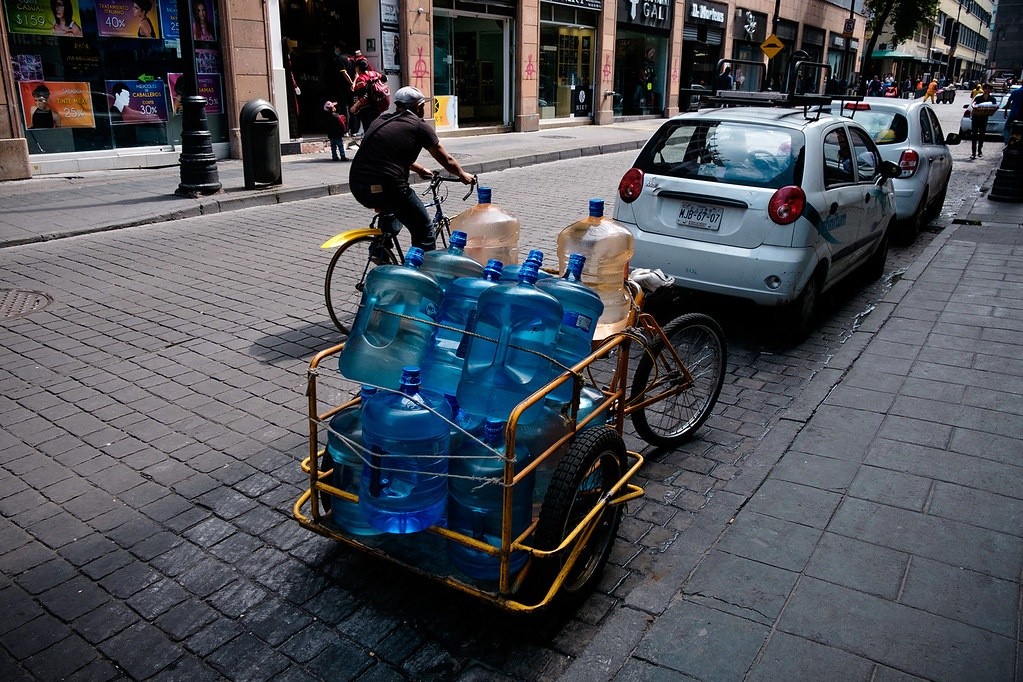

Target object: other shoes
[333,156,339,161]
[341,158,350,161]
[369,244,392,264]
[971,153,982,159]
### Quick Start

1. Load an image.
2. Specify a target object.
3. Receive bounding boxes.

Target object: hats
[324,101,337,111]
[394,86,433,103]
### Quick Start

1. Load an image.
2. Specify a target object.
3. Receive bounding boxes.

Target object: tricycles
[291,266,731,620]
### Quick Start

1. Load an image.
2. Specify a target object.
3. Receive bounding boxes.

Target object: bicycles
[319,170,485,338]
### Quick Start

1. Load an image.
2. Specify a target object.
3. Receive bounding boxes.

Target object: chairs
[713,139,748,169]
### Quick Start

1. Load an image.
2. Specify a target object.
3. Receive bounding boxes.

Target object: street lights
[947,0,972,85]
[969,11,991,89]
[992,26,1008,62]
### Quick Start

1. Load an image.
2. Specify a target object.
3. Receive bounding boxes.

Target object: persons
[639,47,658,106]
[700,66,1023,159]
[349,50,372,137]
[190,0,214,41]
[50,0,82,36]
[349,86,474,266]
[26,85,62,130]
[132,0,156,38]
[323,43,384,163]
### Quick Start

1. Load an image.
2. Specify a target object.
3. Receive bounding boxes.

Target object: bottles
[456,262,564,423]
[557,198,633,342]
[448,422,535,579]
[358,365,453,534]
[535,253,604,402]
[418,229,485,293]
[567,386,609,492]
[326,385,385,536]
[417,259,504,398]
[450,187,519,267]
[501,250,553,285]
[454,408,487,439]
[513,404,571,510]
[338,247,445,390]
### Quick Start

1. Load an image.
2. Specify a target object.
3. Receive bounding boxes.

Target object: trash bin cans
[240,99,283,189]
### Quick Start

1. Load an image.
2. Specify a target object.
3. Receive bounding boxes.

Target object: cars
[609,59,902,338]
[1006,84,1023,96]
[796,96,962,251]
[957,93,1013,140]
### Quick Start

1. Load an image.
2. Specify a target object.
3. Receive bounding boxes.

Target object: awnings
[867,48,948,66]
